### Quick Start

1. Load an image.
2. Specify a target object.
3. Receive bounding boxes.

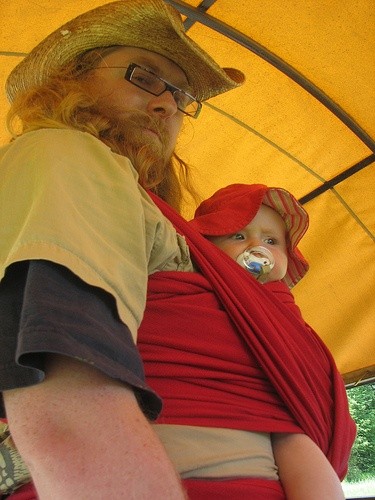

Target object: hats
[188,185,309,290]
[5,0,245,108]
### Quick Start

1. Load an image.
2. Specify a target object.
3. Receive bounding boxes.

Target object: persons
[0,0,286,500]
[185,183,345,500]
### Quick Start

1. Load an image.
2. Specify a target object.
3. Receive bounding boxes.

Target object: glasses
[89,63,202,119]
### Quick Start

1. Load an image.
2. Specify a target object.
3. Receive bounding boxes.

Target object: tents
[0,0,375,384]
